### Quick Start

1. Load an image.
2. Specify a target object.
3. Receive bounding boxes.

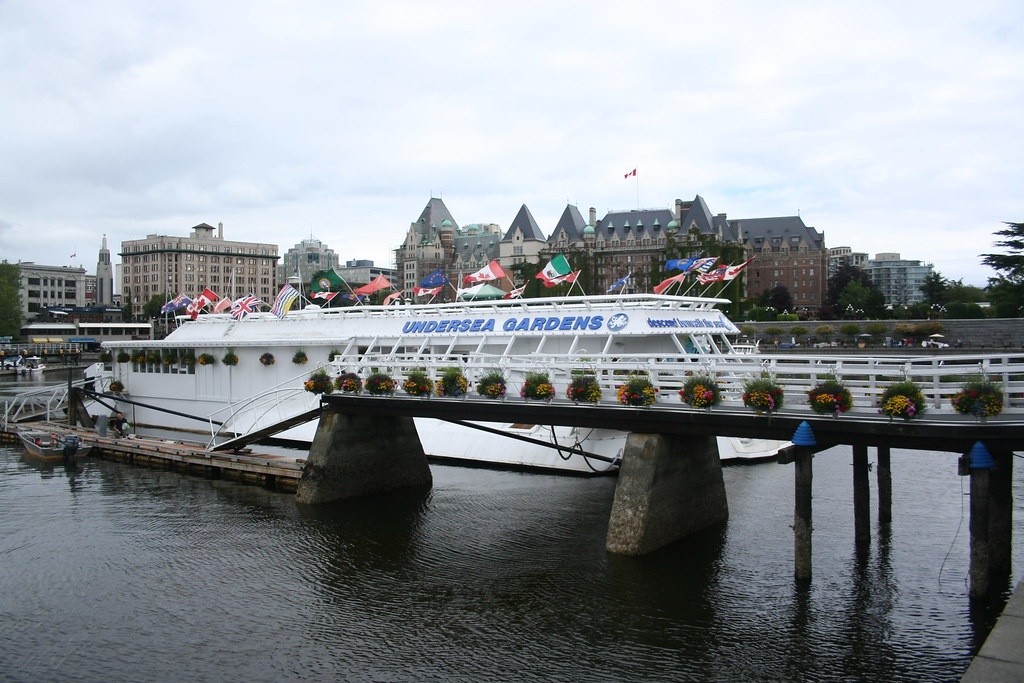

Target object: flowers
[304,369,1008,423]
[109,381,125,392]
[259,353,275,365]
[101,350,238,367]
[292,351,308,366]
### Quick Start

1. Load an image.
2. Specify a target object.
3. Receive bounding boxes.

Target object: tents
[460,282,506,298]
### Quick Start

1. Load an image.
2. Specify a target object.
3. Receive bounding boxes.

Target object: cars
[0,355,24,367]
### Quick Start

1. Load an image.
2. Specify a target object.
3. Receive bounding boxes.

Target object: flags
[696,257,753,286]
[504,285,528,300]
[413,286,445,296]
[664,256,697,272]
[161,293,193,314]
[542,271,579,288]
[70,254,76,257]
[653,271,686,294]
[230,293,264,321]
[624,169,636,179]
[309,267,352,301]
[186,287,233,320]
[606,274,631,293]
[270,284,301,320]
[535,252,572,282]
[464,261,505,284]
[384,292,402,306]
[343,275,391,302]
[687,257,718,274]
[419,269,451,289]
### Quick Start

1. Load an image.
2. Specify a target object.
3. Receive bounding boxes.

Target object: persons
[114,413,128,438]
[892,337,914,348]
[774,338,779,349]
[957,337,962,346]
[60,347,76,353]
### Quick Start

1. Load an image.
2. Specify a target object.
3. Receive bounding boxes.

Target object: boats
[17,356,47,375]
[60,289,811,480]
[15,431,94,462]
